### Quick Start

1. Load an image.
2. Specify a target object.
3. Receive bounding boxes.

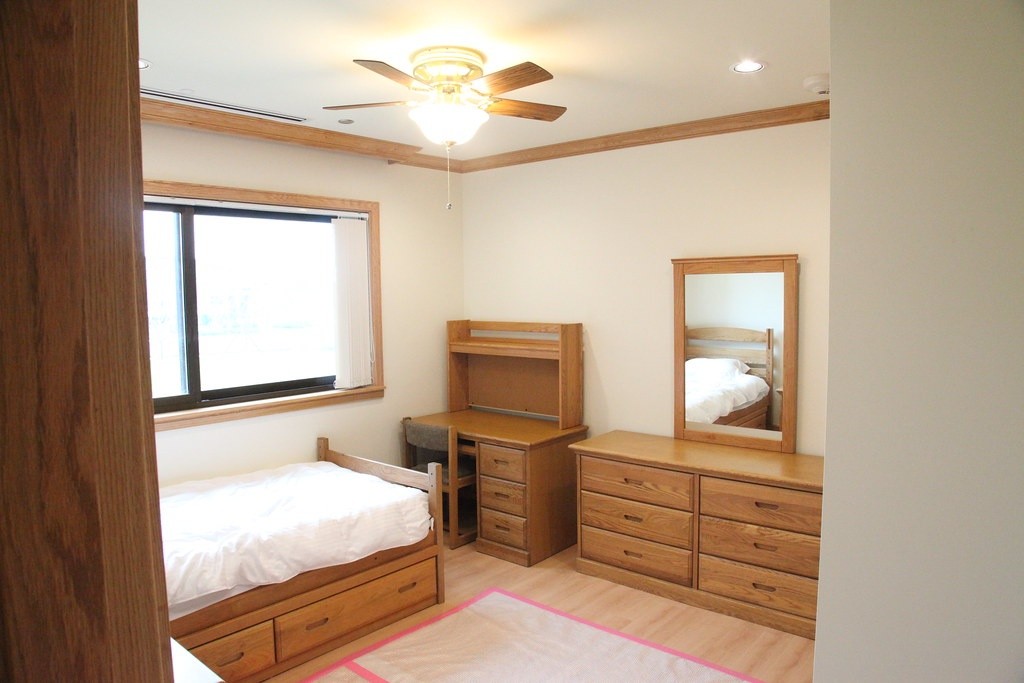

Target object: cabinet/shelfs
[412,318,591,567]
[567,429,824,641]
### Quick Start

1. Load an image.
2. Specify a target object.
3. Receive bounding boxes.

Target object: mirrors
[669,253,801,456]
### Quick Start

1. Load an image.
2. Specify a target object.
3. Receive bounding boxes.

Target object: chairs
[401,417,477,550]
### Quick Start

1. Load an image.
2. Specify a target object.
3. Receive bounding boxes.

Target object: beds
[684,325,774,432]
[157,436,446,683]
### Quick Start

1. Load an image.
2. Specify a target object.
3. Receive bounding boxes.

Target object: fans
[323,44,567,123]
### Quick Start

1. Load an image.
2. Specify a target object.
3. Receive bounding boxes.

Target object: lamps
[407,89,492,212]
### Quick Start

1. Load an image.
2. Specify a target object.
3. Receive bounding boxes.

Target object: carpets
[297,587,763,683]
